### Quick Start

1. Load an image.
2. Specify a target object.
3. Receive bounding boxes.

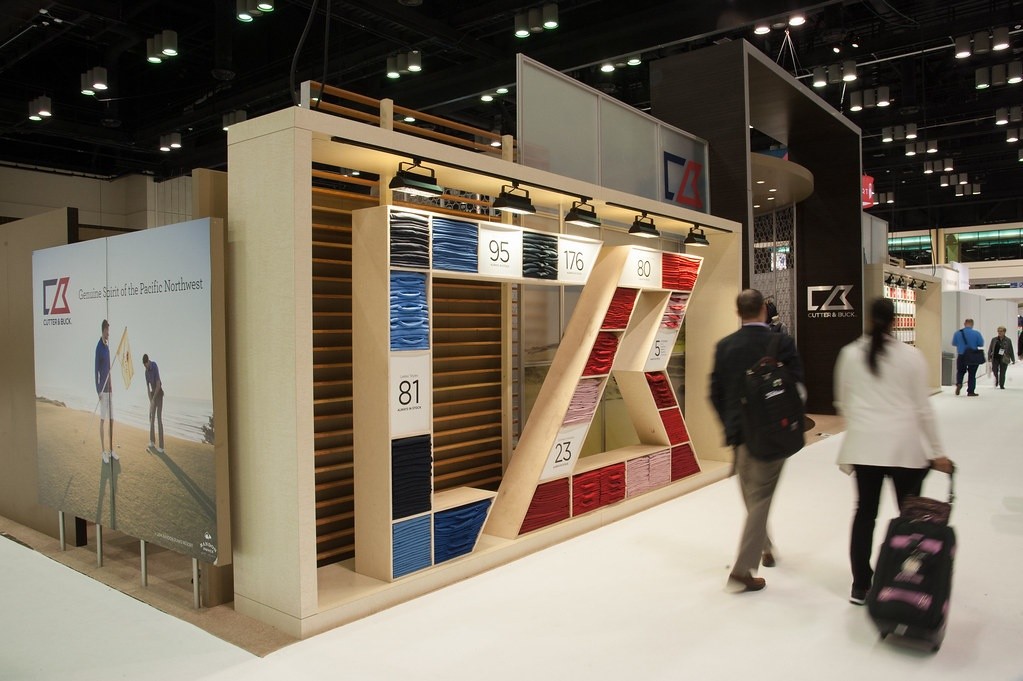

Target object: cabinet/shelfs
[351,204,705,583]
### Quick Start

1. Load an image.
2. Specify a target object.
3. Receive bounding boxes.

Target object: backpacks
[741,324,807,462]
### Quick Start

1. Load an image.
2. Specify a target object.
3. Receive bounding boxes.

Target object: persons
[143,354,164,453]
[94,319,120,464]
[711,288,804,593]
[831,299,949,604]
[952,318,983,396]
[988,326,1015,389]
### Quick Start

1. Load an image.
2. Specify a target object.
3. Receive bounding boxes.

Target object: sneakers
[110,451,120,461]
[102,451,110,464]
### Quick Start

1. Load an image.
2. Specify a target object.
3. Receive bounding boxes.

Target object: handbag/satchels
[1002,354,1011,365]
[898,495,952,526]
[963,349,986,366]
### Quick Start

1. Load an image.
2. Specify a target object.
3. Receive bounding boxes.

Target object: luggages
[868,465,957,652]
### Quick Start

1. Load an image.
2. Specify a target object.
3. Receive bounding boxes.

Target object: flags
[116,328,134,389]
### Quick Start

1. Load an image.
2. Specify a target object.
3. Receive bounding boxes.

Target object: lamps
[628,211,660,239]
[29,96,53,121]
[955,27,1023,162]
[884,274,928,291]
[80,67,108,96]
[386,50,422,79]
[683,224,709,246]
[388,159,443,198]
[223,109,246,131]
[490,129,502,147]
[492,180,537,215]
[564,197,601,228]
[813,60,890,111]
[236,0,275,22]
[516,3,559,38]
[755,13,805,35]
[160,133,182,151]
[600,53,641,72]
[873,123,981,205]
[147,30,179,63]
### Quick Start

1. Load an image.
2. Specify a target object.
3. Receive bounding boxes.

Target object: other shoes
[849,579,872,605]
[728,574,766,593]
[967,392,979,397]
[761,550,776,568]
[148,442,155,448]
[158,448,165,454]
[995,378,998,387]
[999,383,1005,390]
[955,383,962,396]
